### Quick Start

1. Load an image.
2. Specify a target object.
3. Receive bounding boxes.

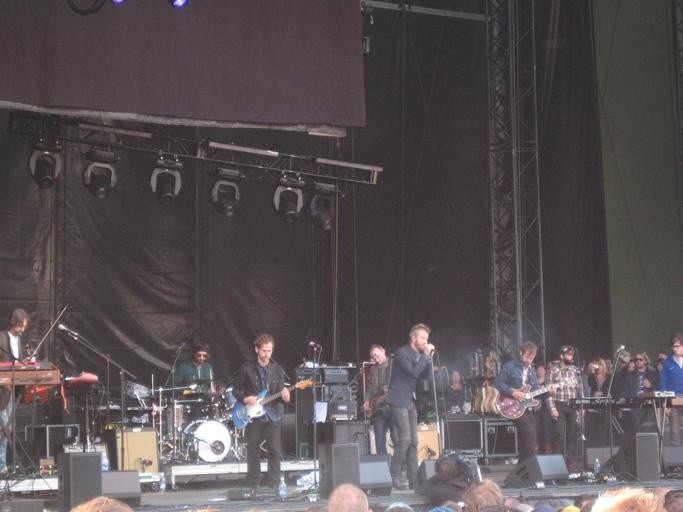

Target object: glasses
[634,358,644,362]
[196,353,208,358]
[671,344,681,347]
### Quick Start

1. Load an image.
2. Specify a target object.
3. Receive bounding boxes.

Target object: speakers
[58,452,102,512]
[629,433,659,482]
[319,443,360,499]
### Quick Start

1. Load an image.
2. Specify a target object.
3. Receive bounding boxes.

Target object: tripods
[164,350,186,465]
[597,354,628,485]
[303,351,319,494]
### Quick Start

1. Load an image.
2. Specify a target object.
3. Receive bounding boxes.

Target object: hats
[193,342,209,352]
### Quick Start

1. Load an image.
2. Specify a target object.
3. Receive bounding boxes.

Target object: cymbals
[162,345,189,350]
[180,378,211,385]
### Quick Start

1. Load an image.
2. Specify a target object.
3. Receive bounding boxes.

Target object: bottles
[594,459,600,474]
[279,478,286,499]
[160,473,165,492]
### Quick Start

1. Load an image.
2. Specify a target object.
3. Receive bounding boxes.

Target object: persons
[0,306,30,474]
[325,482,369,511]
[172,342,216,393]
[493,340,545,490]
[535,365,549,388]
[655,350,666,372]
[464,479,505,512]
[446,368,466,410]
[362,343,398,457]
[232,332,291,487]
[544,343,584,473]
[620,350,658,399]
[385,323,436,495]
[586,354,613,398]
[420,451,478,511]
[659,331,683,446]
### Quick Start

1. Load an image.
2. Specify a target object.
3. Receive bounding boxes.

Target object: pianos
[1,361,61,386]
[570,396,672,408]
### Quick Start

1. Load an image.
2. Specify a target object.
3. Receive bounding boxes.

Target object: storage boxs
[30,420,80,461]
[443,415,483,457]
[483,414,519,459]
[320,418,369,457]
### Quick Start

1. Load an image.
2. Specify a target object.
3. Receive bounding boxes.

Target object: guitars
[463,346,500,417]
[22,342,50,408]
[498,378,581,420]
[230,379,315,430]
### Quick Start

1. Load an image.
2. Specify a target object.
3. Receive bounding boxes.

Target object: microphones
[616,345,625,352]
[309,341,321,348]
[58,324,78,336]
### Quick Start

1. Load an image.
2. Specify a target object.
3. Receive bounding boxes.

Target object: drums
[219,380,237,412]
[180,418,233,464]
